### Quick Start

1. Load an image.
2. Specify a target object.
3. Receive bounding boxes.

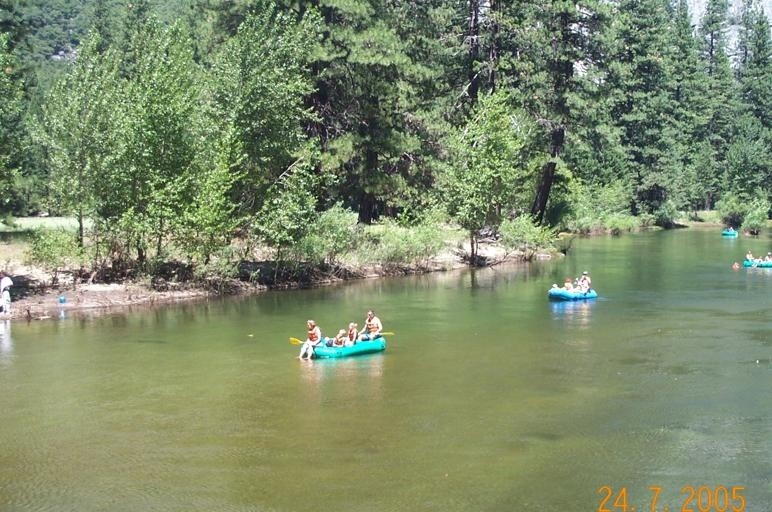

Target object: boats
[721,230,738,236]
[548,288,597,301]
[743,259,772,267]
[305,333,386,359]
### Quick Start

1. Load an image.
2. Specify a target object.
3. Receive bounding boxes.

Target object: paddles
[289,337,305,345]
[378,332,394,336]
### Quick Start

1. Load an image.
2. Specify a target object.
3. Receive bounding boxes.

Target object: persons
[720,225,734,234]
[332,330,345,347]
[0,270,14,314]
[746,250,755,261]
[344,323,358,346]
[563,276,574,292]
[1,319,16,357]
[571,276,589,294]
[297,319,321,360]
[766,252,772,260]
[751,255,763,269]
[358,310,383,341]
[579,270,591,288]
[732,261,741,270]
[746,267,770,288]
